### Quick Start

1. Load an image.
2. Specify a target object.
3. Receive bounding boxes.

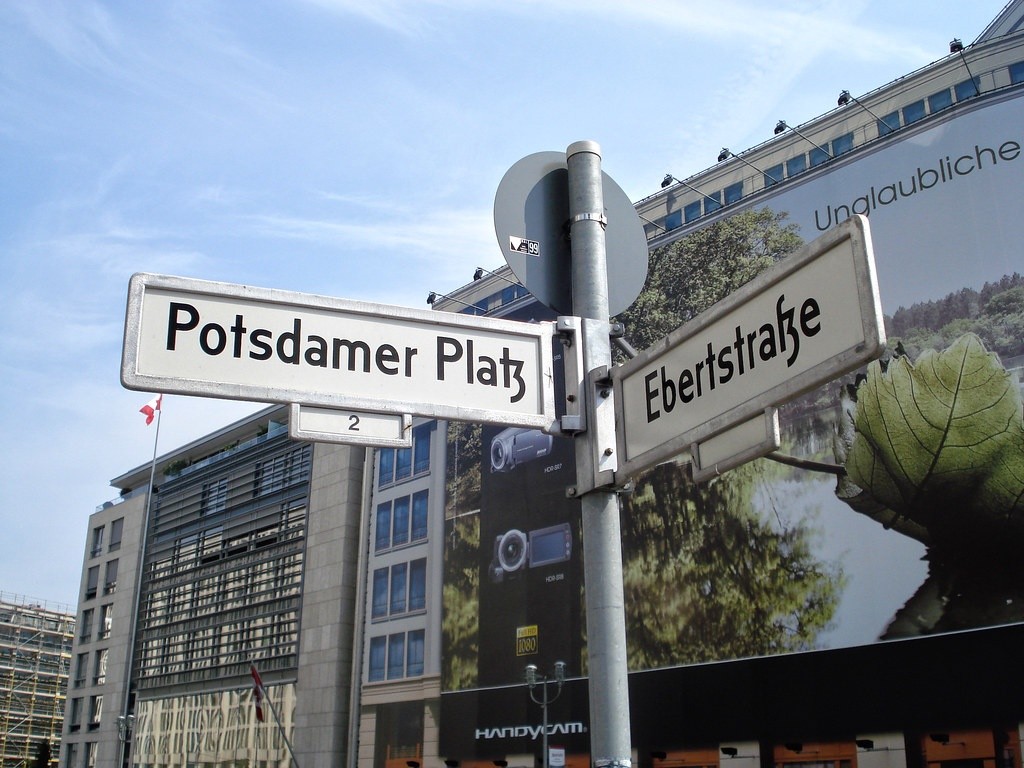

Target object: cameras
[491,424,553,475]
[492,523,573,580]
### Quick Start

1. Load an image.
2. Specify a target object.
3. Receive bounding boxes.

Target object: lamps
[659,172,724,209]
[835,87,895,133]
[925,726,973,753]
[406,760,420,768]
[770,117,835,159]
[645,746,688,764]
[714,145,779,185]
[946,35,981,99]
[443,758,462,768]
[852,733,894,758]
[425,289,488,314]
[492,758,527,768]
[471,265,525,292]
[992,729,1024,746]
[719,742,761,763]
[780,734,822,761]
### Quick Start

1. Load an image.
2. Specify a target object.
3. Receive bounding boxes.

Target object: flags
[139,393,161,425]
[250,665,265,722]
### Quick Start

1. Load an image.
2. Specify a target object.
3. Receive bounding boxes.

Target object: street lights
[118,715,134,768]
[524,662,566,768]
